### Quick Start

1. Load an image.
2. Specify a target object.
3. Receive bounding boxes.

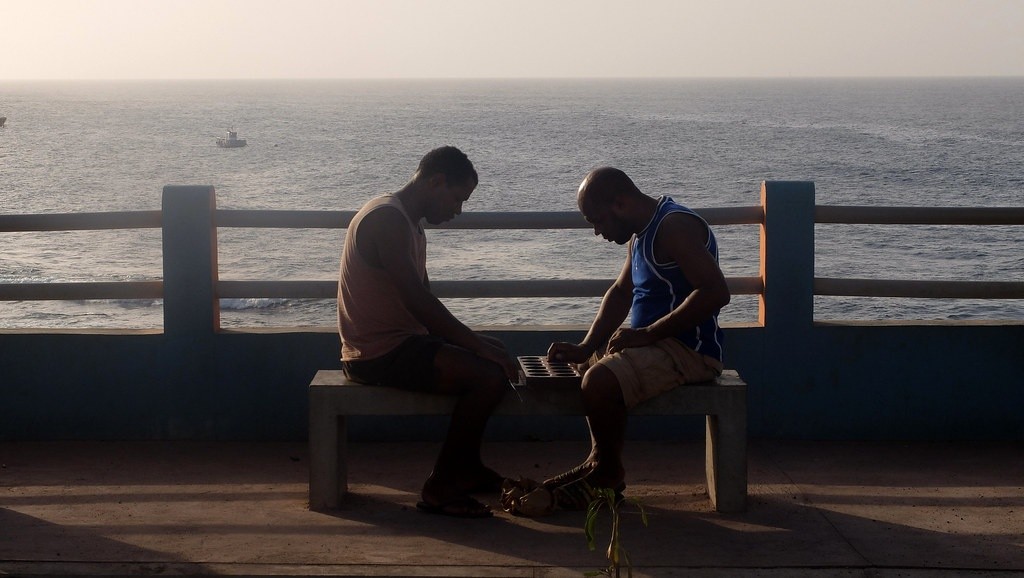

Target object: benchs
[308,370,750,516]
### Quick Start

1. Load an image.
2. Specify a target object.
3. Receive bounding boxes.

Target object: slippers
[417,497,493,517]
[552,476,625,509]
[543,467,582,493]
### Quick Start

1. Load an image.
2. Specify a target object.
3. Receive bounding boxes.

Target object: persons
[539,167,730,511]
[335,145,521,516]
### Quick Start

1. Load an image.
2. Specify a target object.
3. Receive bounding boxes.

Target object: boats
[217,130,247,150]
